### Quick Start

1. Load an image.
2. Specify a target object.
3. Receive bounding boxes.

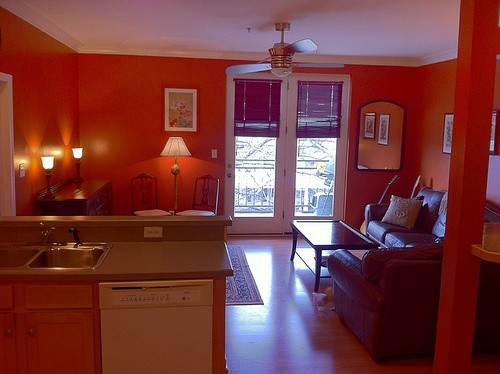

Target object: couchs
[326,186,500,364]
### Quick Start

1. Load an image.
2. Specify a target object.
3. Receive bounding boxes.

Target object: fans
[225,23,344,75]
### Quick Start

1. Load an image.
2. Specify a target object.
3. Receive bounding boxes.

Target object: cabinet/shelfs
[0,280,102,374]
[36,178,112,216]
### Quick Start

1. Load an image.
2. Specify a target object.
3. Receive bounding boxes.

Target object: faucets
[68,227,84,246]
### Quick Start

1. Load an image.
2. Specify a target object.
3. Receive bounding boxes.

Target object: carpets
[226,245,264,306]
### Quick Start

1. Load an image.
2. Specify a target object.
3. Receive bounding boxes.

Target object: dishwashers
[98,279,213,374]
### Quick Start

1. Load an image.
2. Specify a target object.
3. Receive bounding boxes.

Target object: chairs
[175,175,220,216]
[130,173,171,217]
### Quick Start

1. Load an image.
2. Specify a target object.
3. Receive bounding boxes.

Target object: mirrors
[356,100,406,172]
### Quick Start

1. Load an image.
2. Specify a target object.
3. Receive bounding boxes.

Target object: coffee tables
[288,220,380,293]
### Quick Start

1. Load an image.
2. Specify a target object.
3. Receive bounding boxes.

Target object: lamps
[160,136,192,216]
[41,156,55,198]
[71,147,83,188]
[269,56,293,78]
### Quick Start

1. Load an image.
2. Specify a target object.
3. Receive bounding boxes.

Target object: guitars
[410,174,421,199]
[359,174,400,235]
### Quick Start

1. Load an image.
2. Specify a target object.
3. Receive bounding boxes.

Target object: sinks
[0,241,39,271]
[28,241,113,271]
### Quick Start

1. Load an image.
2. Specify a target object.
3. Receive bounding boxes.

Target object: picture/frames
[164,87,197,132]
[364,113,376,140]
[377,114,390,146]
[442,113,454,155]
[489,109,498,156]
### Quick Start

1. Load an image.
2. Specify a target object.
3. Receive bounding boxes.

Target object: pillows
[380,195,424,229]
[432,192,448,236]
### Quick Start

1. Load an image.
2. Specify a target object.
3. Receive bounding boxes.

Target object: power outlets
[143,226,162,238]
